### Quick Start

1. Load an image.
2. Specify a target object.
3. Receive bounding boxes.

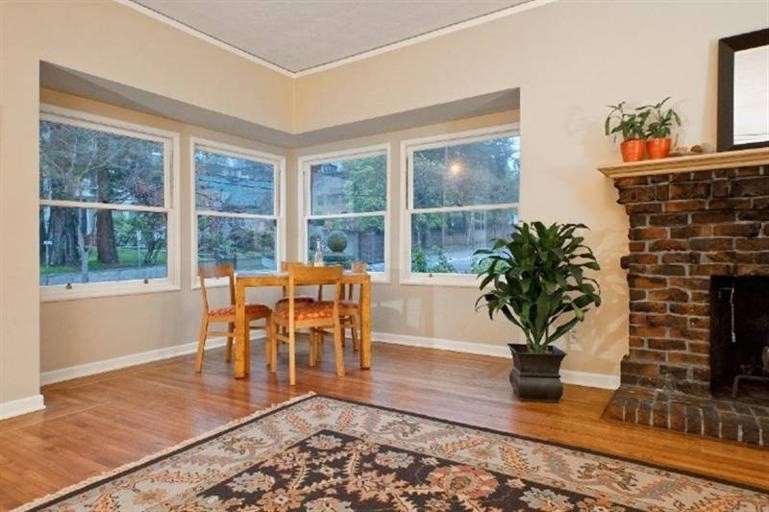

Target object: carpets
[0,389,769,510]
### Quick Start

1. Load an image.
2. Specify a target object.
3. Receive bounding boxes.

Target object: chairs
[195,260,361,387]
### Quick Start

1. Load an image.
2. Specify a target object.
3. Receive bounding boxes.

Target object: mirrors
[715,25,768,153]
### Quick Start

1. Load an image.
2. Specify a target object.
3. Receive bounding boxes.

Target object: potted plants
[605,95,683,164]
[467,216,604,403]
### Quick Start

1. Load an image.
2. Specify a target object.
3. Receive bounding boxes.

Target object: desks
[233,270,371,380]
